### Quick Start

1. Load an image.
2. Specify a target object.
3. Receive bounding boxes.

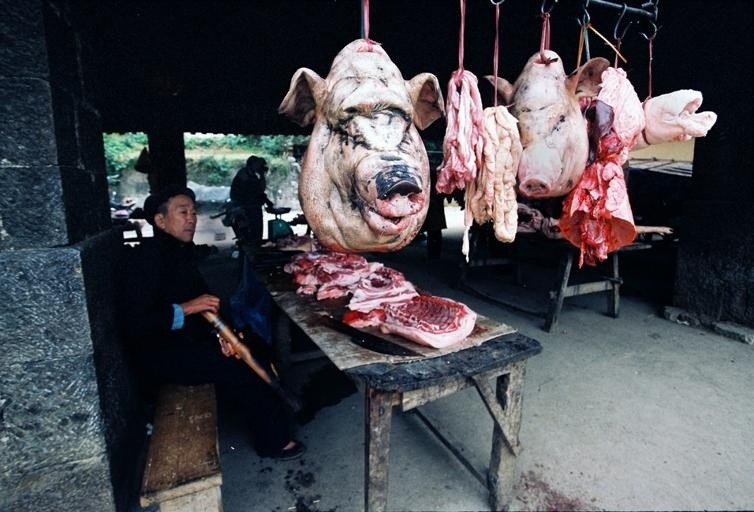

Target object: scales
[265,207,293,240]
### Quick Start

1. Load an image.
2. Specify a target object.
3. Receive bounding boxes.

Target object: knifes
[318,312,425,363]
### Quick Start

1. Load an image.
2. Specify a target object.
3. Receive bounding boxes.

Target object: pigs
[484,50,611,199]
[277,38,447,255]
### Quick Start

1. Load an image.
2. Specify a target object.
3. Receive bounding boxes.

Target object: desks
[423,227,672,334]
[248,228,545,512]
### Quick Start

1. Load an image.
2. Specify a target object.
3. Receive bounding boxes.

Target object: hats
[144,188,195,226]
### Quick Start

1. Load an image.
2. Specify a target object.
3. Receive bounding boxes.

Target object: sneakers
[257,440,307,460]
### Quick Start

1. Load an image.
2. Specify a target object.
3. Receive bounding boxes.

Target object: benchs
[138,379,228,512]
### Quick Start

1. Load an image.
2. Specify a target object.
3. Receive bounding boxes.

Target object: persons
[116,156,306,460]
